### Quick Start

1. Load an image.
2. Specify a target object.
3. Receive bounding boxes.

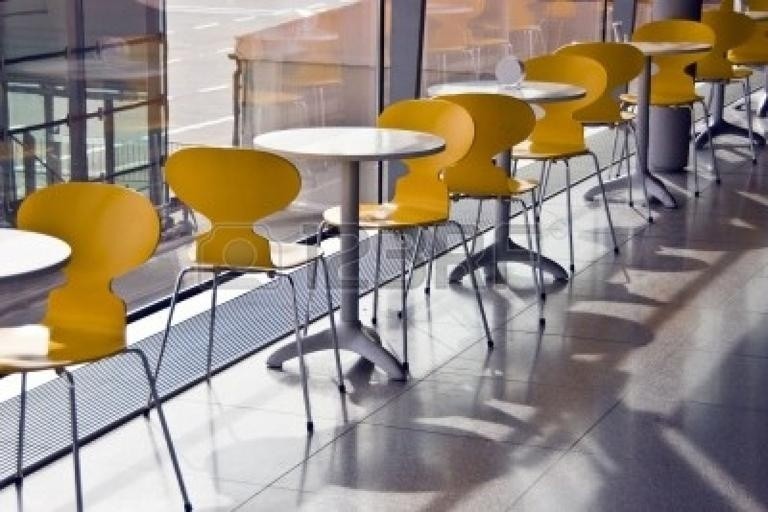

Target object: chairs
[2,1,767,512]
